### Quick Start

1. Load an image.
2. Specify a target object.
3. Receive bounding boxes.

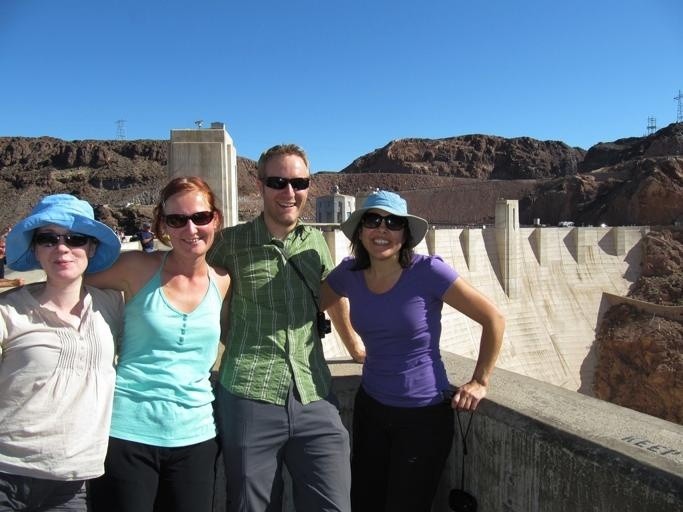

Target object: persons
[0,194,123,512]
[204,146,366,512]
[112,224,119,237]
[139,222,156,255]
[317,191,504,511]
[120,229,126,242]
[85,178,230,510]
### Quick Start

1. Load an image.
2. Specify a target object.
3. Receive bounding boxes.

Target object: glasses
[35,232,89,247]
[166,211,213,228]
[262,177,310,189]
[363,213,406,230]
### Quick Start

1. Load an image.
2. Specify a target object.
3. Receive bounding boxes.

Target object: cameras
[448,489,477,512]
[318,311,331,338]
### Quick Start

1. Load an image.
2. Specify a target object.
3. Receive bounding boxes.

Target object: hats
[5,194,121,274]
[340,190,429,249]
